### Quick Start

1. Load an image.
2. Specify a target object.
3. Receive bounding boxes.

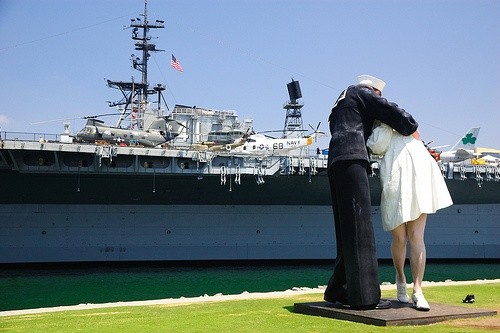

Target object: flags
[132,99,139,130]
[171,54,183,72]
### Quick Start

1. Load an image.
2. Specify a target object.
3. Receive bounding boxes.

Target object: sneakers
[412,292,431,311]
[396,273,410,304]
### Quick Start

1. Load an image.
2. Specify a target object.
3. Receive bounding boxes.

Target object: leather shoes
[323,293,393,310]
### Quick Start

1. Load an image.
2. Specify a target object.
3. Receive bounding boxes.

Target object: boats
[0,0,500,269]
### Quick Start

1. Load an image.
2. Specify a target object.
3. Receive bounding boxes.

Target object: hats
[358,75,386,93]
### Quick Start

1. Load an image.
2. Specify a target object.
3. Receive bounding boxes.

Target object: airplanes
[417,125,500,167]
[227,121,326,157]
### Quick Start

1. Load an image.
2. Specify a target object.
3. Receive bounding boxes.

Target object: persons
[367,123,454,311]
[324,75,421,309]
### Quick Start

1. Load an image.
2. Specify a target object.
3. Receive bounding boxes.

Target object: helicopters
[31,112,184,149]
